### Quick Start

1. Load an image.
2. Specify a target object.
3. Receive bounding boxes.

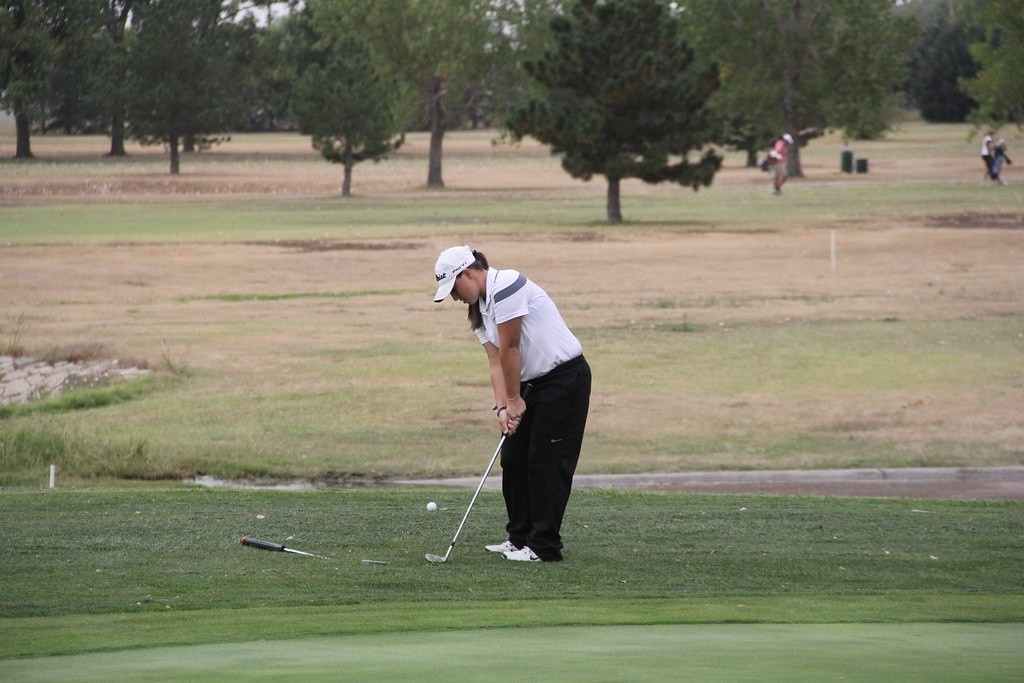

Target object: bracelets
[497,406,507,416]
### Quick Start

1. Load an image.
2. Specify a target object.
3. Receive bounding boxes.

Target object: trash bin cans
[856,159,868,174]
[841,150,852,173]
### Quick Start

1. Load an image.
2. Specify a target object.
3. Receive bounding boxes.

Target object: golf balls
[427,501,437,512]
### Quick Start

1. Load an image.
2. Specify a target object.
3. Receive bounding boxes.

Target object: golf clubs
[423,383,534,563]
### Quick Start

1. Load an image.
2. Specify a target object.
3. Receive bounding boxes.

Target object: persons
[772,133,793,193]
[433,246,592,563]
[982,128,1007,186]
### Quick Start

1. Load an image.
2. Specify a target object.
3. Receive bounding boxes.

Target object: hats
[783,133,793,143]
[433,245,475,303]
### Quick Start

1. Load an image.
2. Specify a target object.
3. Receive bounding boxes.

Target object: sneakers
[484,541,520,554]
[501,545,542,561]
